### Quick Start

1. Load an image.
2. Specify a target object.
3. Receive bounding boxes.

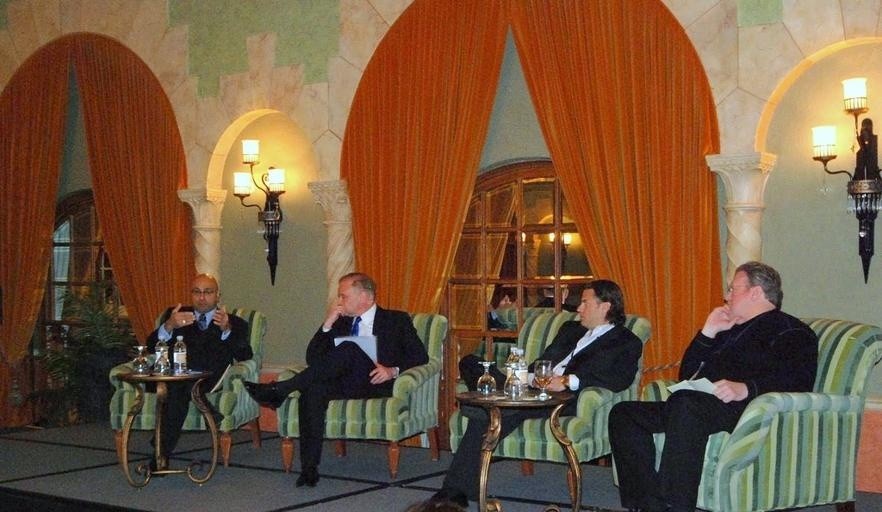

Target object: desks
[458,390,580,512]
[115,372,218,486]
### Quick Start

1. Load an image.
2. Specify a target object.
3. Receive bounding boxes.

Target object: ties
[198,314,207,331]
[352,317,361,336]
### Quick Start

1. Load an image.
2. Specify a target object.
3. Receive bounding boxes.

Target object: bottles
[514,349,528,400]
[155,336,169,357]
[506,347,517,362]
[174,335,188,375]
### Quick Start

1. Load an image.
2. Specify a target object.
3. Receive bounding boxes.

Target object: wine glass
[153,346,170,376]
[504,362,524,400]
[534,360,552,400]
[132,345,149,376]
[478,360,498,395]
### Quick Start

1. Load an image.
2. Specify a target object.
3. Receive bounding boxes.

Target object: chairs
[476,343,514,367]
[109,306,268,469]
[277,313,449,477]
[450,314,650,477]
[612,319,882,511]
[496,308,567,331]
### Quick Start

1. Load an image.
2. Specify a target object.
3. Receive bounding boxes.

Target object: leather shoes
[418,492,470,510]
[458,401,518,422]
[296,466,317,487]
[243,380,285,409]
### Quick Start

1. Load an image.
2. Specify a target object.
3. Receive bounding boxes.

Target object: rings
[182,318,186,326]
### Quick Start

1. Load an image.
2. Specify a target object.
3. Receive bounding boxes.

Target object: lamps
[812,77,882,284]
[550,232,572,268]
[233,139,286,285]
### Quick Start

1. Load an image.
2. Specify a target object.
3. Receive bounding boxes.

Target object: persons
[144,272,253,474]
[608,261,817,512]
[407,280,642,510]
[243,272,429,486]
[533,286,578,312]
[494,291,512,309]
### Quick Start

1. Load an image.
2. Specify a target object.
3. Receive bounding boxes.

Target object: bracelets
[561,375,570,387]
